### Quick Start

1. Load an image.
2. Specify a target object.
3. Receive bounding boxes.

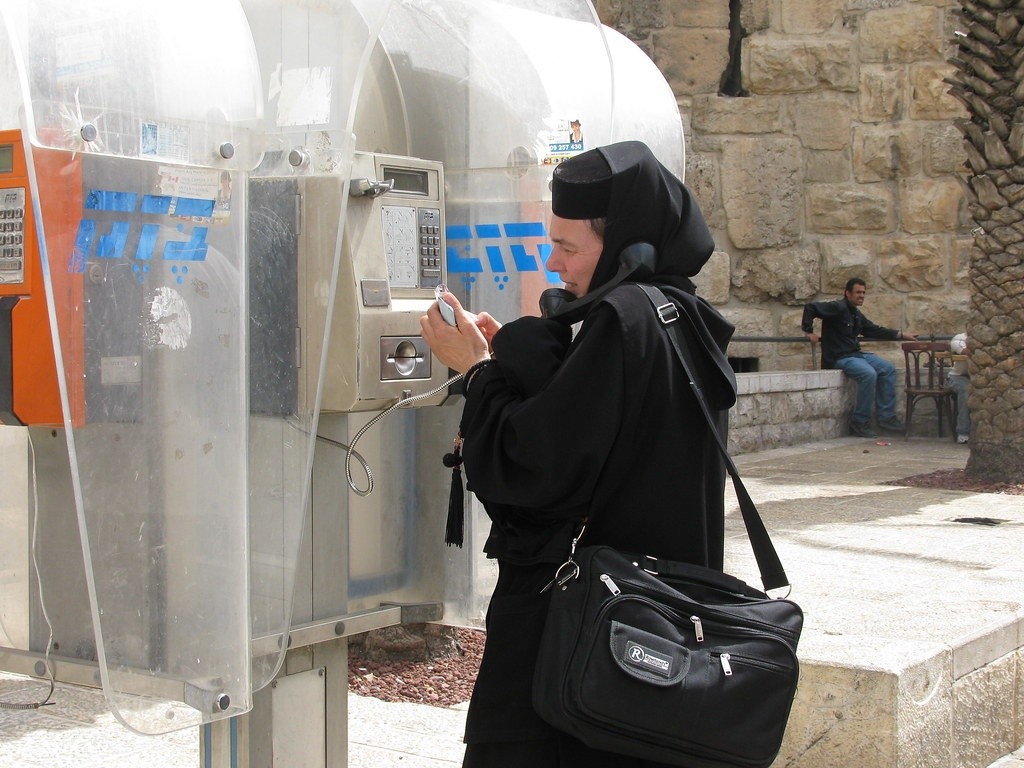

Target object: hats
[551,141,649,219]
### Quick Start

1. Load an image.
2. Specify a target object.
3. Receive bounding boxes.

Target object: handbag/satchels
[532,547,804,768]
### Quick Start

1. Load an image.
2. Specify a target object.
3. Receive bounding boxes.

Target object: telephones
[535,241,659,326]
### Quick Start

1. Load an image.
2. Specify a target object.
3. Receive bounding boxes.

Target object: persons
[419,139,738,767]
[800,276,920,439]
[947,320,970,444]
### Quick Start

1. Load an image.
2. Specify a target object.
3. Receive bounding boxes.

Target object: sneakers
[877,416,905,432]
[850,421,878,438]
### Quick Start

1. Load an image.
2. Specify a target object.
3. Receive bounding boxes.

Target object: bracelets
[461,358,490,399]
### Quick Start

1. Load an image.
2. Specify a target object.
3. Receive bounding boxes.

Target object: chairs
[902,341,958,443]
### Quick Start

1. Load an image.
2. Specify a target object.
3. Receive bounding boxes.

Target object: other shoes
[957,432,969,443]
